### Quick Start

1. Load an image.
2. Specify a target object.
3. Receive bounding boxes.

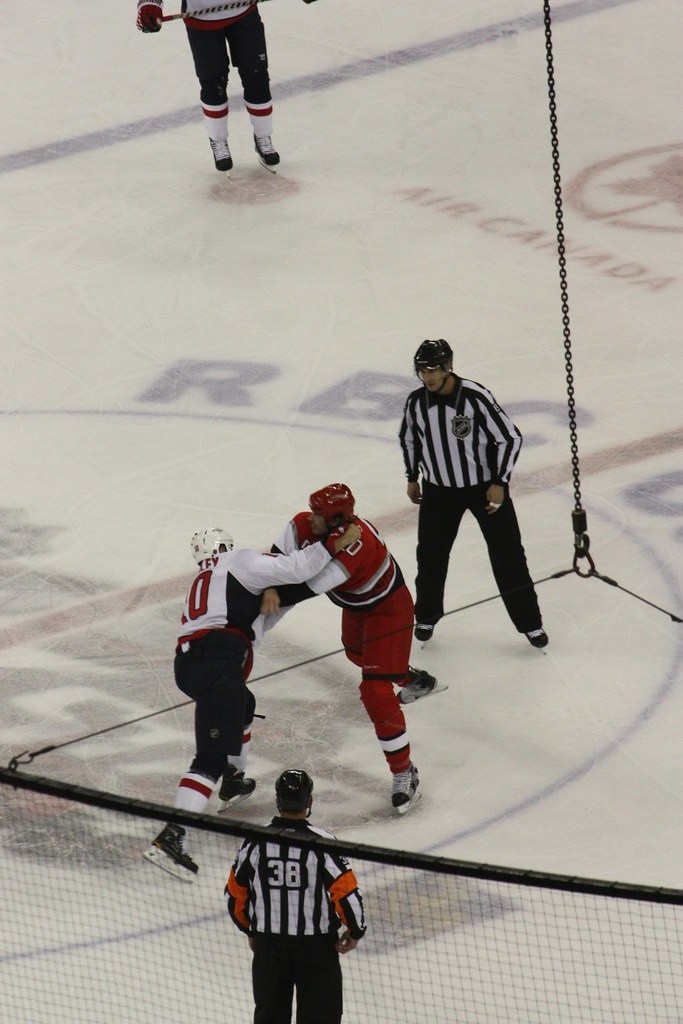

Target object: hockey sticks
[162,0,270,22]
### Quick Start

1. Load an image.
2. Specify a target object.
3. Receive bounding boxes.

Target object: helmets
[189,527,233,565]
[413,338,453,371]
[274,769,313,802]
[308,482,355,527]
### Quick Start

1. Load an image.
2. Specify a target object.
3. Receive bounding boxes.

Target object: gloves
[136,0,164,34]
[321,520,365,559]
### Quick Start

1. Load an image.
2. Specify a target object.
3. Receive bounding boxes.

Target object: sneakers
[142,823,199,882]
[391,762,423,814]
[525,628,549,648]
[209,137,233,180]
[397,665,438,705]
[414,622,434,641]
[217,764,256,812]
[253,133,280,174]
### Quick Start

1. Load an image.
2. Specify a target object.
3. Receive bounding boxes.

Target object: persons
[142,522,361,884]
[259,484,448,814]
[398,339,550,658]
[224,769,367,1023]
[135,0,322,178]
